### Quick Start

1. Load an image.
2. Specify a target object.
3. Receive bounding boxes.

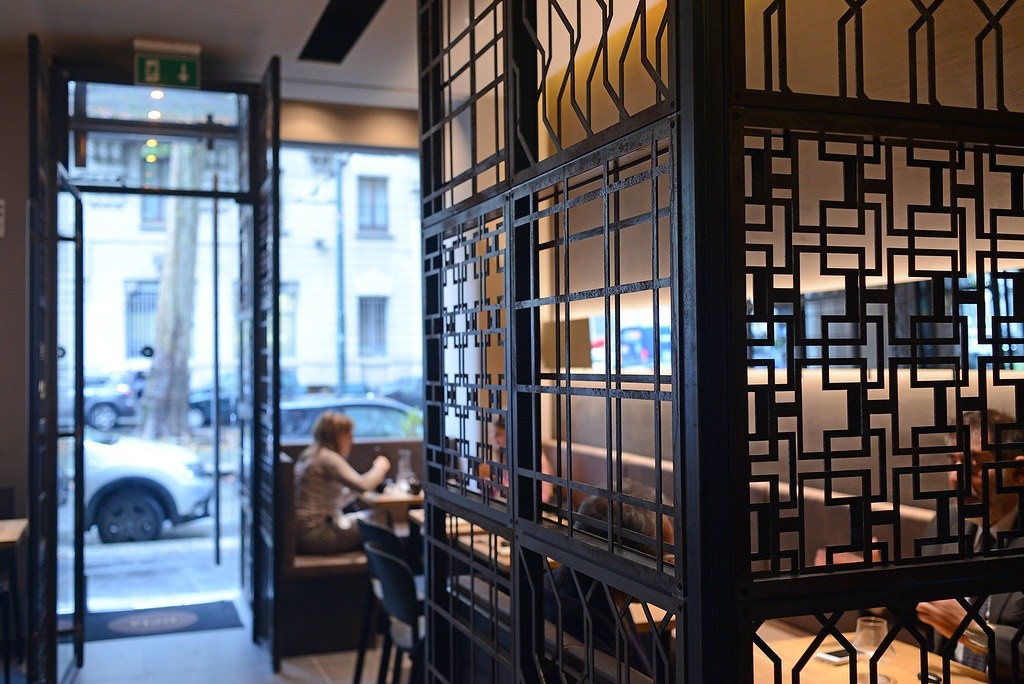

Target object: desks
[359,481,993,684]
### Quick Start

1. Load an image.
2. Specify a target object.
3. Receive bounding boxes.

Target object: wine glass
[854,617,890,684]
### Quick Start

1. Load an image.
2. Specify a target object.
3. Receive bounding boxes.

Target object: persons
[476,409,554,503]
[537,482,678,684]
[292,411,391,556]
[815,409,1024,684]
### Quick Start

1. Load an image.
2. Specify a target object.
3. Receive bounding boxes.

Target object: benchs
[534,438,940,642]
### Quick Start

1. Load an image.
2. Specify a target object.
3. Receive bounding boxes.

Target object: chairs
[276,452,473,684]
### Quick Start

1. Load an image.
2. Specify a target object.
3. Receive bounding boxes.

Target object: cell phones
[815,647,867,665]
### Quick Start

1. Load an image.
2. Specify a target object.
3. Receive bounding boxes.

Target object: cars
[589,326,674,368]
[56,425,214,548]
[56,358,426,444]
